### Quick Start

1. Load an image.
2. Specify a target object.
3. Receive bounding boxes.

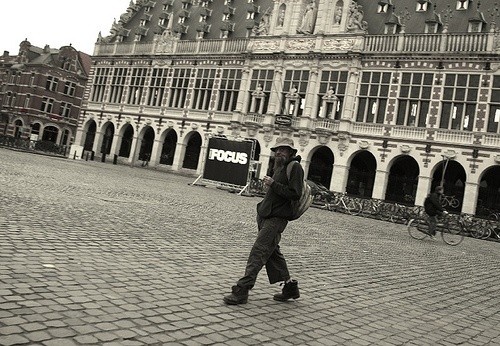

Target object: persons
[424,186,449,240]
[222,138,304,305]
[278,10,285,27]
[335,7,342,24]
[296,0,318,34]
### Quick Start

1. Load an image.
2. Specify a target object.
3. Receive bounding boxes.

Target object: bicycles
[441,194,460,208]
[266,182,500,240]
[407,209,466,246]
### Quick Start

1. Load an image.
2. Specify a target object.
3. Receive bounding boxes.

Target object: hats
[270,136,298,154]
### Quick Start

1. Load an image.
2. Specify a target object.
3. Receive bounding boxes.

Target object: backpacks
[289,181,315,220]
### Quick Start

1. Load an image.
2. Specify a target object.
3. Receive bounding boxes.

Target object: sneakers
[273,279,300,302]
[222,285,250,305]
